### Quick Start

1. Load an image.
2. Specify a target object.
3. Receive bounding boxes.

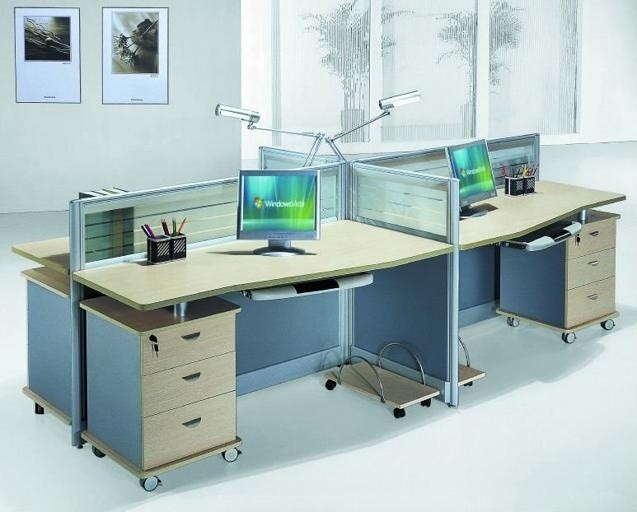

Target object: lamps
[215,90,423,168]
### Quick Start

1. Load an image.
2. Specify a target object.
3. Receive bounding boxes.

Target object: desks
[10,133,626,491]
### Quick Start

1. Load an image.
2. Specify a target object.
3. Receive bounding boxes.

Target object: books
[78,186,136,264]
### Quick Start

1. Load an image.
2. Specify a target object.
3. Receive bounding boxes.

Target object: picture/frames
[14,6,169,105]
